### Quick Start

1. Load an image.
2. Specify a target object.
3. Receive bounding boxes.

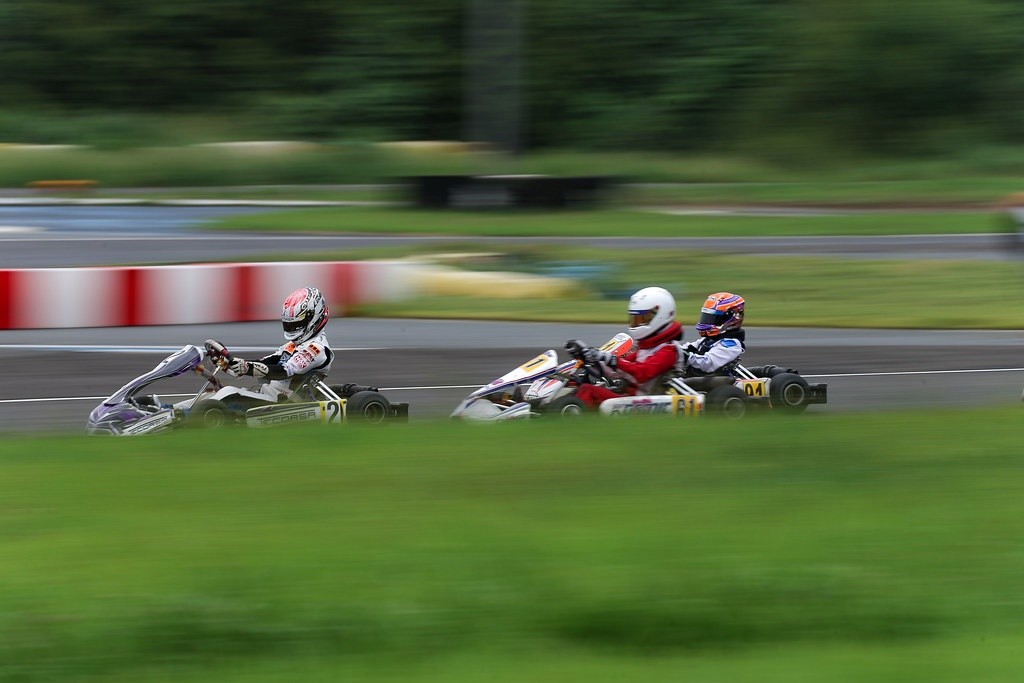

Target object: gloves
[564,338,586,361]
[581,346,617,368]
[210,354,230,373]
[227,357,269,378]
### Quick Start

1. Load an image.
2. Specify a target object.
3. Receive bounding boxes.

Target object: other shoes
[512,386,525,403]
[152,392,174,410]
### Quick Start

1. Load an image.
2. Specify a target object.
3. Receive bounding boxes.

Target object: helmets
[281,286,329,345]
[627,287,676,340]
[695,292,745,337]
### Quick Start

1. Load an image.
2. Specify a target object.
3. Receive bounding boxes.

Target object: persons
[515,286,686,412]
[682,293,745,376]
[134,288,333,411]
